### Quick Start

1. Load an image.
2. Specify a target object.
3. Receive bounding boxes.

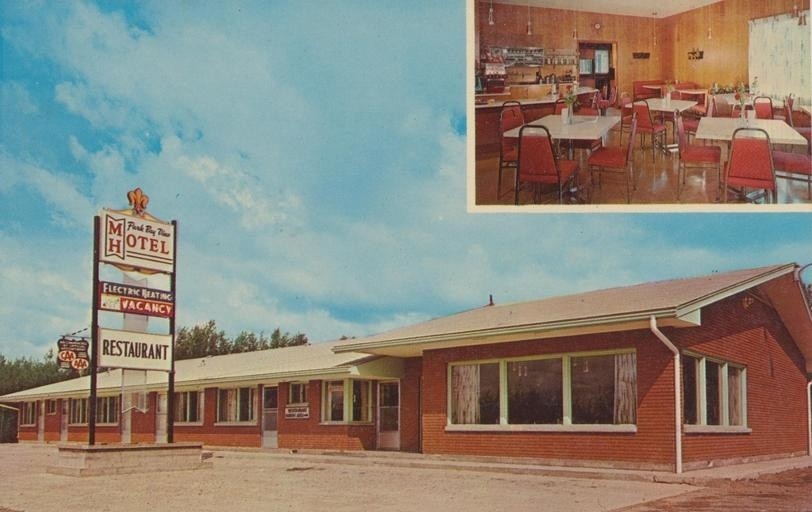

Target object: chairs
[495,77,811,205]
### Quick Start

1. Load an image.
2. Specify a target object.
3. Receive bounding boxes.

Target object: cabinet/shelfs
[478,46,580,95]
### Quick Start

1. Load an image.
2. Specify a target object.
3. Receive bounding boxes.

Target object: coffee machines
[484,62,508,94]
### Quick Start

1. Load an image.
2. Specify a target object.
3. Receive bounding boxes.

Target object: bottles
[546,56,578,64]
[561,108,569,125]
[539,73,555,83]
[708,81,733,94]
[558,72,572,81]
[675,80,679,84]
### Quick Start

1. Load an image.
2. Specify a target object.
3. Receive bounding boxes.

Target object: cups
[488,99,496,105]
[662,95,668,108]
[747,110,756,124]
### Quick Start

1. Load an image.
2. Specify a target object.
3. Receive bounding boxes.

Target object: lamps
[706,1,713,41]
[526,3,532,36]
[570,9,578,42]
[487,0,495,26]
[651,12,658,48]
